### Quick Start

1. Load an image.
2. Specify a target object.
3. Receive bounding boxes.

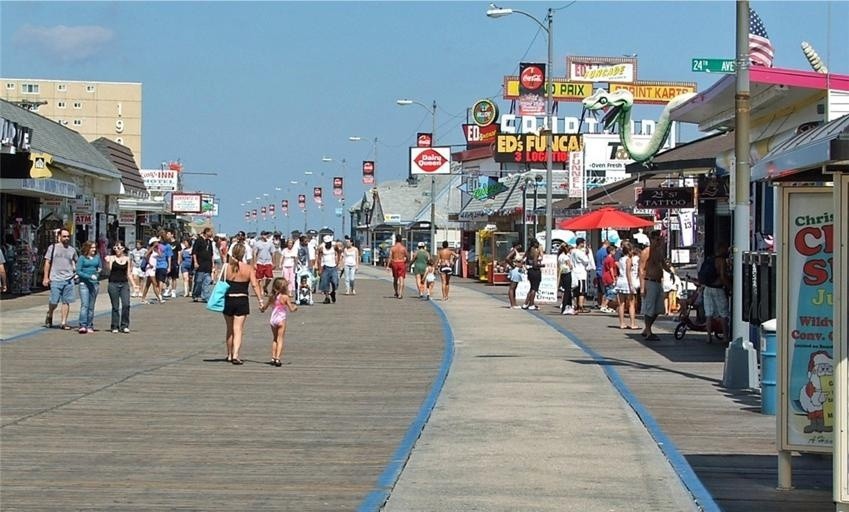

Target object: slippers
[262,286,269,296]
[621,324,660,343]
[226,356,244,365]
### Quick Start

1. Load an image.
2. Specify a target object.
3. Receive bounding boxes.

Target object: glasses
[112,246,124,250]
[62,234,70,238]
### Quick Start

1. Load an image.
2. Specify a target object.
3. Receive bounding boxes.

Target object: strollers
[295,268,315,306]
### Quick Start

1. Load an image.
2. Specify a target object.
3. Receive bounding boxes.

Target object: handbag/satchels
[36,270,51,290]
[206,262,231,312]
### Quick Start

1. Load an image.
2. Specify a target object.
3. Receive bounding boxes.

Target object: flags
[747,7,775,70]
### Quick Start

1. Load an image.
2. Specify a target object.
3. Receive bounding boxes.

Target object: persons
[263,277,298,367]
[703,241,732,346]
[40,228,79,331]
[0,248,9,293]
[386,234,411,300]
[103,239,140,334]
[76,240,104,334]
[435,241,459,301]
[410,241,431,297]
[129,226,361,305]
[421,259,437,300]
[222,244,263,365]
[505,230,678,342]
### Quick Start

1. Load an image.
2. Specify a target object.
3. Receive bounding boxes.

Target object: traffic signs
[691,59,736,72]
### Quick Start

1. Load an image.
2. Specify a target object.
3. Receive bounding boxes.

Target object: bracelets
[259,299,264,303]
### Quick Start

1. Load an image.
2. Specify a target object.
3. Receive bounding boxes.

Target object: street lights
[363,201,373,246]
[397,97,438,257]
[486,2,555,253]
[241,178,308,233]
[321,156,347,237]
[305,171,324,228]
[348,136,379,185]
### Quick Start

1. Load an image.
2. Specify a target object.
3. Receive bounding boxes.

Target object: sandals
[270,358,282,367]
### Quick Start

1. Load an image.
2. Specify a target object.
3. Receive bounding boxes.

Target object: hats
[322,235,334,243]
[418,241,425,248]
[148,236,160,246]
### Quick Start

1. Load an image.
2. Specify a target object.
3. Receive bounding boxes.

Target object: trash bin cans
[760,318,777,416]
[362,247,372,264]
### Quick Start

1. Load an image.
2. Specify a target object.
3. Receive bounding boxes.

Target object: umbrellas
[558,206,658,242]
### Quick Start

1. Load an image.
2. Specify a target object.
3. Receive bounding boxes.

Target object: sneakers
[133,288,208,305]
[393,291,449,302]
[510,303,617,315]
[322,291,336,304]
[345,289,356,295]
[45,311,130,333]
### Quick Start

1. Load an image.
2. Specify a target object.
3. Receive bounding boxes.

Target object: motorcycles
[672,276,729,340]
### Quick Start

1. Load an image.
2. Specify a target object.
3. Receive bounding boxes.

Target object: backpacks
[696,255,726,285]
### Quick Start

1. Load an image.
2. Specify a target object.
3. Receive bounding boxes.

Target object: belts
[644,277,663,284]
